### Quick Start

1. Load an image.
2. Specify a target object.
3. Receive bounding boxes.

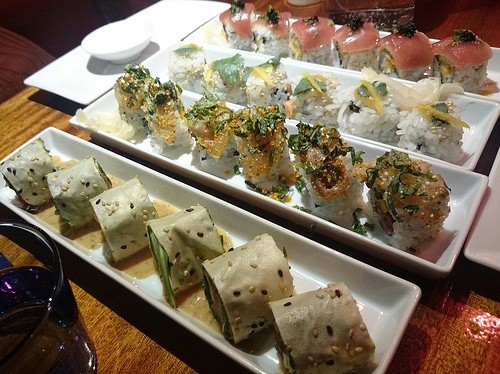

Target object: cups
[322,0,415,32]
[0,220,97,374]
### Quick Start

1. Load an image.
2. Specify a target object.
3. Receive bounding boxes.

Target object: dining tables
[0,1,498,374]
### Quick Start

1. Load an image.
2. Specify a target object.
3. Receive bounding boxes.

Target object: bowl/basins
[81,19,153,65]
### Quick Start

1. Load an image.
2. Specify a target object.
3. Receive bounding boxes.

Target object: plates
[69,70,488,277]
[0,126,421,374]
[463,148,500,271]
[182,11,500,105]
[23,0,232,105]
[133,40,500,170]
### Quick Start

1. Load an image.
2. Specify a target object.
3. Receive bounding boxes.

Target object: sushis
[143,203,225,308]
[266,281,376,374]
[114,0,492,253]
[90,176,160,265]
[0,139,59,209]
[201,234,295,345]
[45,155,113,230]
